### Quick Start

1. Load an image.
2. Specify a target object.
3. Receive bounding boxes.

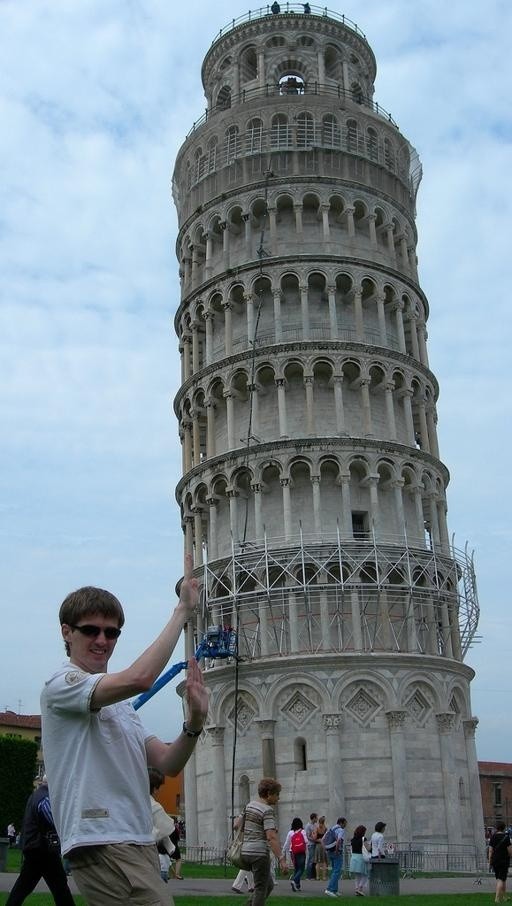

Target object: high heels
[175,876,184,881]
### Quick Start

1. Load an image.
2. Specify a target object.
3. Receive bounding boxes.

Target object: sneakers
[324,890,338,898]
[354,892,359,896]
[291,880,297,892]
[231,887,244,894]
[355,890,365,896]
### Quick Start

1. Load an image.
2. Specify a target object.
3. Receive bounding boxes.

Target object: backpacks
[323,827,340,852]
[291,830,306,855]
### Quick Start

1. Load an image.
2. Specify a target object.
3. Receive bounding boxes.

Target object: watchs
[182,721,203,738]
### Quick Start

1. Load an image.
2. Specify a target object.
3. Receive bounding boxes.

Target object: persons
[311,816,327,880]
[7,821,15,847]
[489,821,512,904]
[148,769,176,883]
[169,813,186,881]
[305,813,318,880]
[232,779,282,906]
[322,817,347,898]
[39,554,209,906]
[231,869,254,894]
[279,818,309,892]
[5,774,75,904]
[370,822,386,859]
[349,825,371,897]
[14,831,22,845]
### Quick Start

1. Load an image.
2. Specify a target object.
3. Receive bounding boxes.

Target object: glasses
[75,624,121,639]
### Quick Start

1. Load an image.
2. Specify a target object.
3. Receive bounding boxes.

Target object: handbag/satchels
[490,835,508,867]
[226,805,251,873]
[44,830,60,851]
[362,837,372,865]
